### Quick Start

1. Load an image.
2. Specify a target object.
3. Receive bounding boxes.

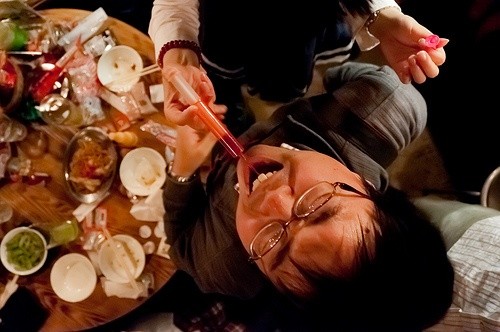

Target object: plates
[97,45,143,93]
[120,147,167,196]
[62,127,117,204]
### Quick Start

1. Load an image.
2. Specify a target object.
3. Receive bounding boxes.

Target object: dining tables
[1,8,212,332]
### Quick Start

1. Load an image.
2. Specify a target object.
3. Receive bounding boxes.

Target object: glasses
[247,182,374,263]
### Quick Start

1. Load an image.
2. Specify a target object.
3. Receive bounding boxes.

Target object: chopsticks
[102,63,161,86]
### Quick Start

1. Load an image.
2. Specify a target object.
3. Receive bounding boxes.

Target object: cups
[0,114,27,143]
[29,219,78,249]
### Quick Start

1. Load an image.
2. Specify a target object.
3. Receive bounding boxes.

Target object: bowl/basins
[0,227,48,275]
[51,253,97,303]
[98,235,146,283]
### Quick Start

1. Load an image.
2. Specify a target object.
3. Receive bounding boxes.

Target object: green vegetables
[4,231,44,270]
